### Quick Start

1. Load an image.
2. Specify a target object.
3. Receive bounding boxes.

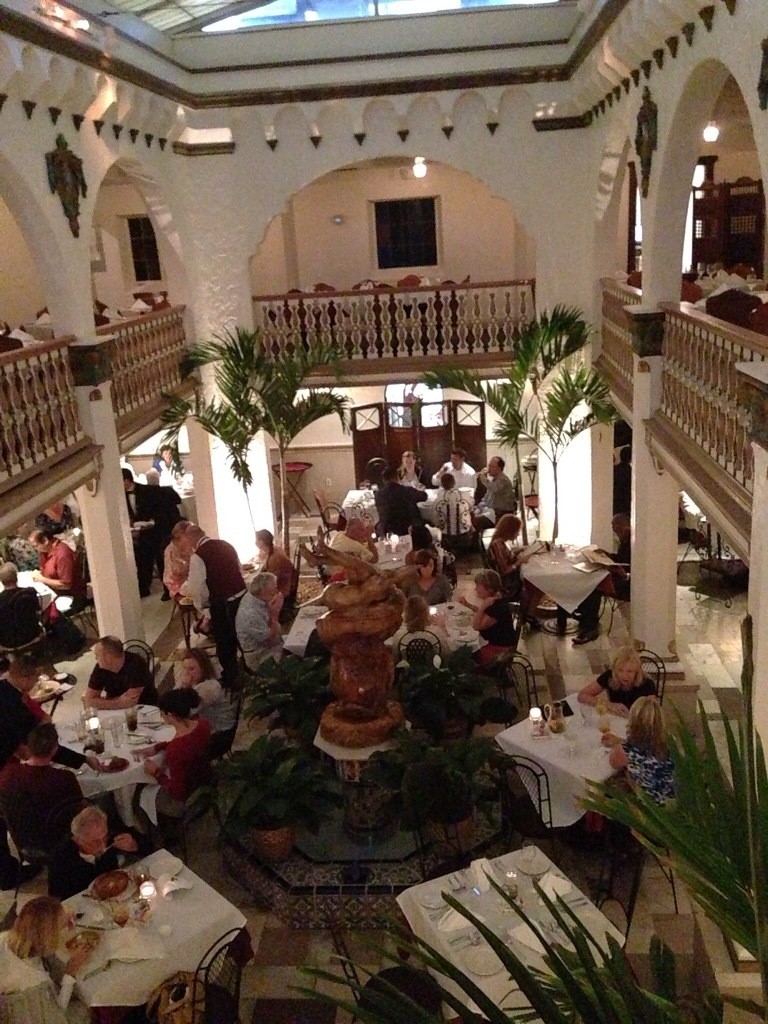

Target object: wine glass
[387,532,399,561]
[564,698,612,766]
[475,467,490,479]
[522,840,537,893]
[697,262,723,281]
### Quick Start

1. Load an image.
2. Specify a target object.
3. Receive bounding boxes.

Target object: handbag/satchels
[145,971,206,1024]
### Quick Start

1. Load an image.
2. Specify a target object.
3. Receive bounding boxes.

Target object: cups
[125,709,138,731]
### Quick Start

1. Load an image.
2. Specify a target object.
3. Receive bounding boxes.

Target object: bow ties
[126,489,136,496]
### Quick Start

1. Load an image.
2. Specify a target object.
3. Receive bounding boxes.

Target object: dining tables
[13,568,59,609]
[366,533,413,569]
[342,490,473,523]
[41,850,247,1024]
[54,704,175,827]
[518,543,610,637]
[397,849,628,1022]
[284,606,449,660]
[492,694,633,830]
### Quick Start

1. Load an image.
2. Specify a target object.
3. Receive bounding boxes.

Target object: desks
[271,462,313,519]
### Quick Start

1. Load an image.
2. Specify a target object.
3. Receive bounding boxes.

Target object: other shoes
[15,864,41,885]
[162,591,171,601]
[139,589,151,599]
[573,629,599,645]
[522,613,542,630]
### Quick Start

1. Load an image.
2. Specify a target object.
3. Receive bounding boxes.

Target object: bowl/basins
[92,870,131,901]
[29,680,60,701]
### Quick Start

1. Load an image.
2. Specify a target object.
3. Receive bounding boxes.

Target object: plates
[418,854,550,976]
[178,600,194,605]
[149,857,182,879]
[64,762,87,777]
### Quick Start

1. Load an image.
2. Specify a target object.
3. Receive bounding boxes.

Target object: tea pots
[543,700,565,738]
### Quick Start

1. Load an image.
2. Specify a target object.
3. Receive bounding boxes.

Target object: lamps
[334,216,342,224]
[0,1,738,151]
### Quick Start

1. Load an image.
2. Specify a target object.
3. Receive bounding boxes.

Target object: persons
[488,514,540,626]
[473,458,514,531]
[614,446,631,516]
[431,448,476,488]
[153,446,183,474]
[572,512,631,644]
[577,644,678,851]
[375,467,427,536]
[397,451,431,488]
[0,469,297,1024]
[678,508,707,550]
[325,520,514,676]
[433,474,476,536]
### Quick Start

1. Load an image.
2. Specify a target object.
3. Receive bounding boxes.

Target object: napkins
[156,873,193,901]
[470,857,503,891]
[437,905,486,933]
[509,917,551,955]
[530,873,572,904]
[140,705,160,716]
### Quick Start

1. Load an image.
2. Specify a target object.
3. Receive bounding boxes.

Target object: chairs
[1,490,732,1023]
[624,263,768,333]
[0,275,471,359]
